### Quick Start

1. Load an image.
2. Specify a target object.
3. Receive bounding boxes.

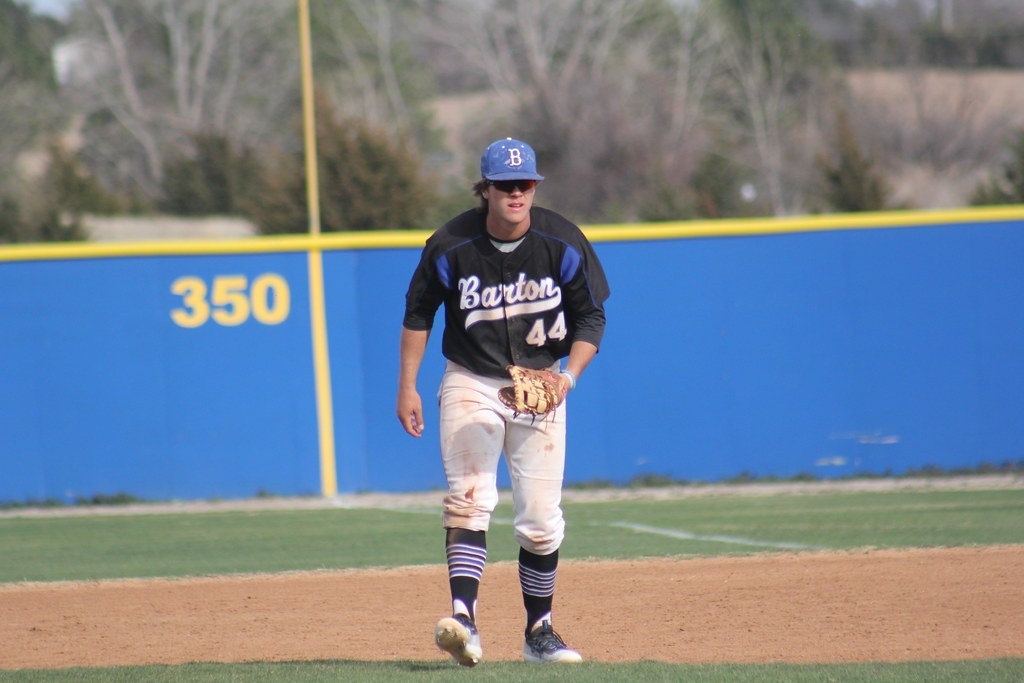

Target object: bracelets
[560,370,576,390]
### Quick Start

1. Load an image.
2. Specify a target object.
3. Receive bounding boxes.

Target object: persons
[396,138,611,667]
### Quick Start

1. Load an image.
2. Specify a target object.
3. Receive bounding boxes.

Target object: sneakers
[434,614,483,666]
[523,620,581,664]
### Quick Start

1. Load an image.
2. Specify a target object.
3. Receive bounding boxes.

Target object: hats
[480,137,544,182]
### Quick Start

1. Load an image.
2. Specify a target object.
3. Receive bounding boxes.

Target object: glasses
[486,180,534,193]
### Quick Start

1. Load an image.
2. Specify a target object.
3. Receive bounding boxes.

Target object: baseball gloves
[498,365,571,415]
